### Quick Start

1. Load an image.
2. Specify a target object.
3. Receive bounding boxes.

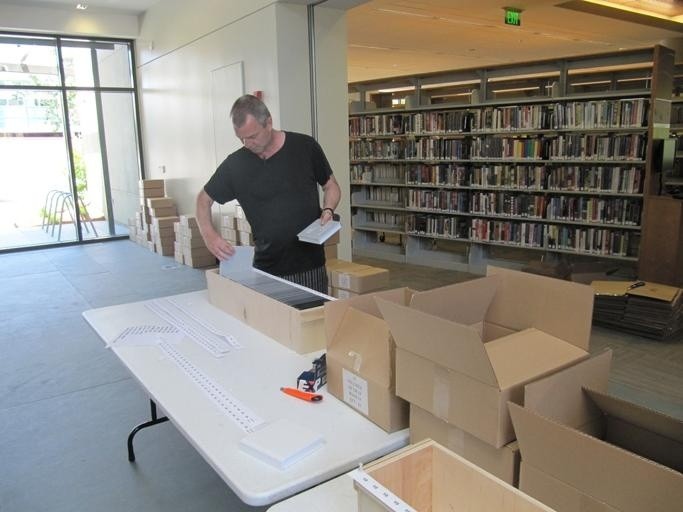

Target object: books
[297,219,342,248]
[474,97,649,257]
[349,110,474,239]
[217,244,330,310]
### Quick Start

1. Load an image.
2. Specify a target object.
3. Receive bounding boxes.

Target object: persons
[193,93,342,295]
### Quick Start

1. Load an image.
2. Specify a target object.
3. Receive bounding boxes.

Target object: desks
[78,288,407,511]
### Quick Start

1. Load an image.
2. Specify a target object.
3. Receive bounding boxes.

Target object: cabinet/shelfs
[346,45,673,264]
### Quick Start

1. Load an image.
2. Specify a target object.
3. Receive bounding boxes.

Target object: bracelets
[320,206,340,221]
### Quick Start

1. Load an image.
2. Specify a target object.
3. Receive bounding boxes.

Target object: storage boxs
[320,232,392,301]
[501,347,681,511]
[405,402,520,489]
[375,267,596,451]
[325,288,424,435]
[125,179,251,269]
